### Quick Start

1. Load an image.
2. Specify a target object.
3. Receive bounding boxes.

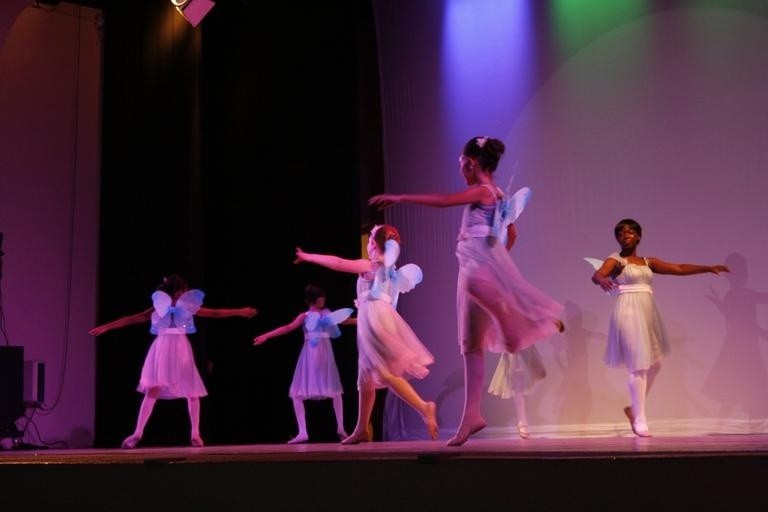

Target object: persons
[583,220,730,436]
[486,317,565,441]
[252,284,357,445]
[291,222,440,445]
[87,277,259,448]
[366,135,565,446]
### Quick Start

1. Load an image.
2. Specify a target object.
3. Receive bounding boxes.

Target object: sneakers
[518,422,530,438]
[624,405,652,438]
[122,433,141,448]
[447,419,487,446]
[337,428,348,440]
[287,435,308,444]
[341,432,370,445]
[191,435,203,446]
